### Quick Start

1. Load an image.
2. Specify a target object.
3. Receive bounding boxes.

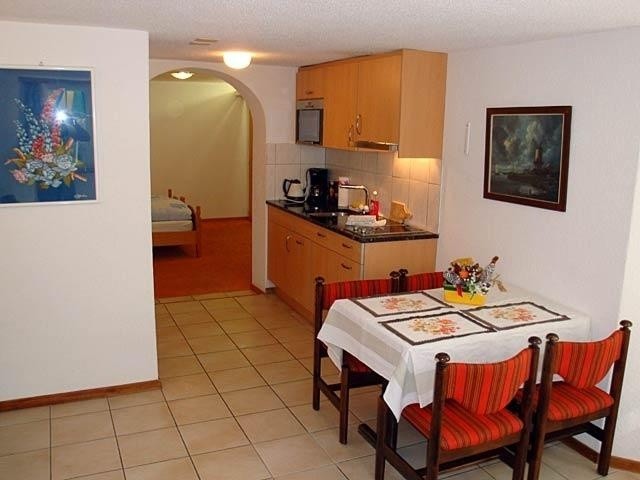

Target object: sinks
[305,210,361,229]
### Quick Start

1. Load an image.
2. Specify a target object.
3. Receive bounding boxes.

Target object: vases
[443,285,487,307]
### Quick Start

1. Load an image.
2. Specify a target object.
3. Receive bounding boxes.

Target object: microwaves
[296,99,323,146]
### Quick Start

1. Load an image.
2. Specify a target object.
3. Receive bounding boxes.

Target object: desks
[314,280,592,480]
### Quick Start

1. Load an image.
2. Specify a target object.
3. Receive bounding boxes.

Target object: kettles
[283,179,306,200]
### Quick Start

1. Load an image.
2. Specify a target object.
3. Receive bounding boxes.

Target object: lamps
[222,50,252,71]
[171,69,197,81]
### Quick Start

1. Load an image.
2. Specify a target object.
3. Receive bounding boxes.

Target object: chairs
[306,273,413,446]
[404,258,458,289]
[387,334,541,478]
[502,321,632,480]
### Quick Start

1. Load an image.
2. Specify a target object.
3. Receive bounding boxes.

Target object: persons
[25,83,90,202]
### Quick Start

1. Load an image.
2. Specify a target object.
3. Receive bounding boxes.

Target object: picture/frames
[0,63,102,206]
[482,103,571,213]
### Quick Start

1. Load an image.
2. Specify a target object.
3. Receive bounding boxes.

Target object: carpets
[151,218,253,298]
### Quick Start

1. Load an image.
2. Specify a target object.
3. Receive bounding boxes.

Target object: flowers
[439,258,505,294]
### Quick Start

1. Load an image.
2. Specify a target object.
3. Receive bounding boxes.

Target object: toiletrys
[338,185,349,210]
[369,190,380,220]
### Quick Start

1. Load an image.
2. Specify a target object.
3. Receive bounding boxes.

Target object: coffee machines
[305,168,328,210]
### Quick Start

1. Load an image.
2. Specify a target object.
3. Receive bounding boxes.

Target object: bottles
[370,191,378,220]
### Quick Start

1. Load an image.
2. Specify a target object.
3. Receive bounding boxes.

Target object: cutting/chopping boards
[389,202,411,224]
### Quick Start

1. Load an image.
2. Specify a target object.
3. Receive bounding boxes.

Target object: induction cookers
[343,224,428,236]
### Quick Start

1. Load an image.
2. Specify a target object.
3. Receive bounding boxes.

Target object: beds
[151,188,204,258]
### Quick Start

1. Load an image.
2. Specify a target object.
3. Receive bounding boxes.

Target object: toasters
[338,183,369,210]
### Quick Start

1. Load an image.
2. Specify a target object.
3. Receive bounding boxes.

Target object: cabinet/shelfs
[295,64,327,100]
[319,51,446,159]
[263,201,439,330]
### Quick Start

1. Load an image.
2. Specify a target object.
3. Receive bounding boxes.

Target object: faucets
[339,184,368,215]
